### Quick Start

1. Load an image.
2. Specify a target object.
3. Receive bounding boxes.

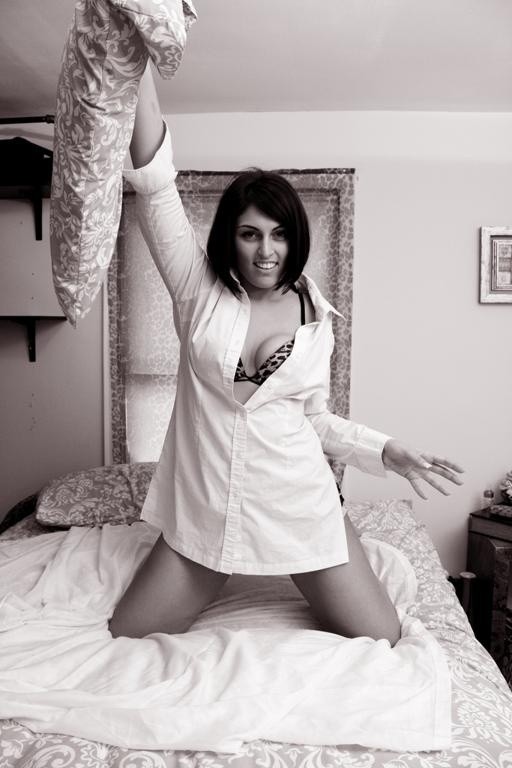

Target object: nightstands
[466,531,512,683]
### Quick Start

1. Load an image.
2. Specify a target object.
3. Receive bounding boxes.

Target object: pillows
[49,0,198,328]
[35,461,159,525]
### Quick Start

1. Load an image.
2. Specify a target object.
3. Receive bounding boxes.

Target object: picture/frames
[480,226,512,304]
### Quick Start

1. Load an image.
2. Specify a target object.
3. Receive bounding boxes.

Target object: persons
[106,52,467,648]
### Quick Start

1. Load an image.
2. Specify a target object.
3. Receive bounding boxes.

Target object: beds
[0,497,512,768]
